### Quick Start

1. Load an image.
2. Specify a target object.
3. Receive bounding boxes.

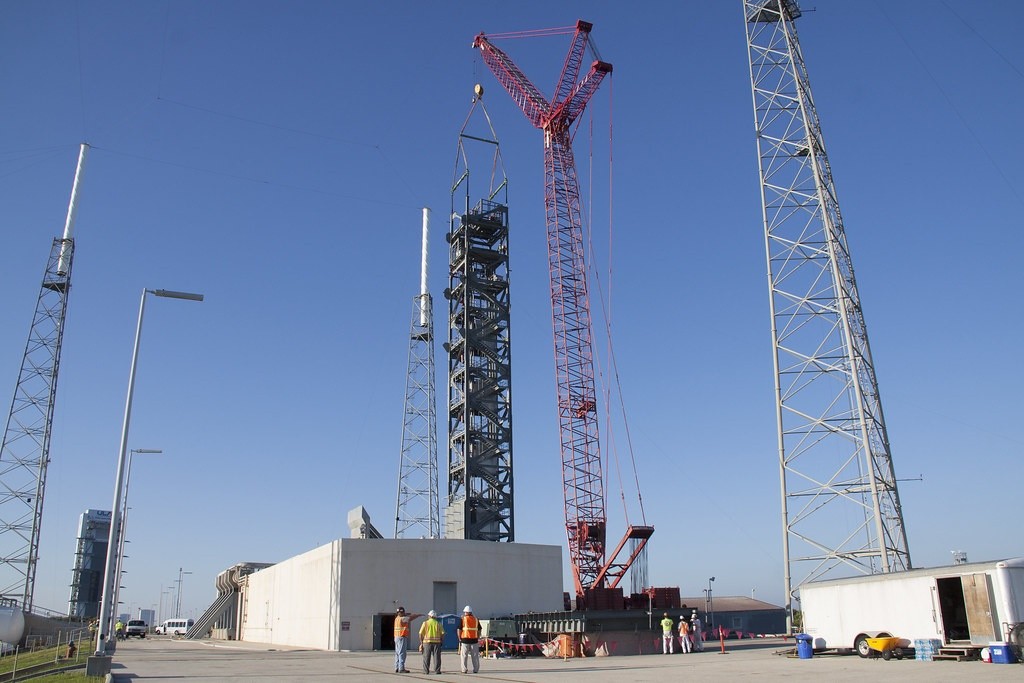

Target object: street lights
[176,568,193,619]
[752,589,756,600]
[94,286,205,656]
[107,448,162,643]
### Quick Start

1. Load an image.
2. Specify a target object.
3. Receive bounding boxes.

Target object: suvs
[123,619,149,639]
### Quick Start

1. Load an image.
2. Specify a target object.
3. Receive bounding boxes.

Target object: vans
[155,618,194,636]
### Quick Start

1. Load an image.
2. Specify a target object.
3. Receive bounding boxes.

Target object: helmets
[680,615,685,620]
[396,607,405,613]
[663,612,668,617]
[462,605,473,613]
[427,610,437,618]
[691,613,697,619]
[692,609,697,614]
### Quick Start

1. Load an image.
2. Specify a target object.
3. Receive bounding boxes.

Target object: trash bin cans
[795,633,813,659]
[437,614,462,650]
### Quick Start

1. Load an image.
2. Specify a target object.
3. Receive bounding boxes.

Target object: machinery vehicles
[470,19,684,611]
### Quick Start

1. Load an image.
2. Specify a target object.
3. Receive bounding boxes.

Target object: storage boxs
[988,641,1015,664]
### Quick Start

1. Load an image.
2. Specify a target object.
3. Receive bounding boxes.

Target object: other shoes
[461,672,467,674]
[399,669,410,674]
[435,671,442,674]
[422,672,429,675]
[473,671,478,673]
[395,668,399,673]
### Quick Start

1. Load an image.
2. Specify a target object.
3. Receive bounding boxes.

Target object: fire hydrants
[66,640,77,659]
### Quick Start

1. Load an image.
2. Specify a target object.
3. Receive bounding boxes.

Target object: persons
[393,607,425,673]
[419,609,446,675]
[678,616,691,653]
[116,620,125,640]
[457,606,482,673]
[88,619,100,641]
[690,614,704,652]
[661,613,674,654]
